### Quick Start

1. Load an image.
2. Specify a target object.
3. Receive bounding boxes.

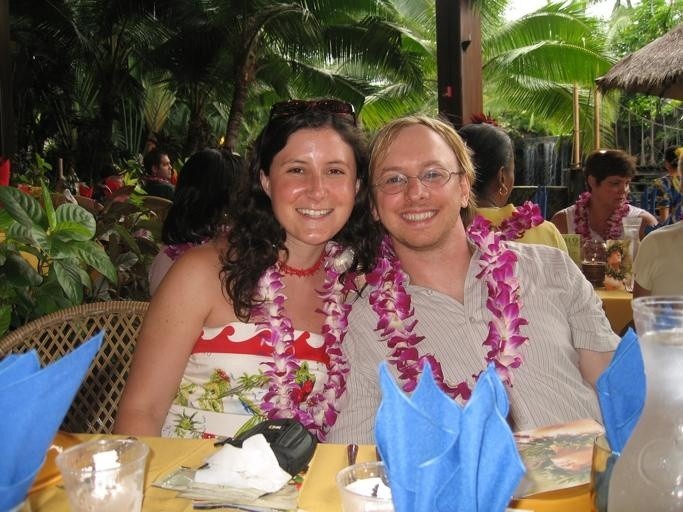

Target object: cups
[593,435,619,509]
[336,459,395,511]
[55,435,150,511]
[581,263,606,288]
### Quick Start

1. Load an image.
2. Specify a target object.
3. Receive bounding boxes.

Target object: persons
[112,99,386,440]
[550,149,658,290]
[324,114,622,444]
[620,220,683,337]
[85,163,127,198]
[148,149,250,297]
[457,123,568,253]
[644,145,683,235]
[141,148,175,201]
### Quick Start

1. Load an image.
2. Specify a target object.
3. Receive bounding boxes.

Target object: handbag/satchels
[214,419,317,479]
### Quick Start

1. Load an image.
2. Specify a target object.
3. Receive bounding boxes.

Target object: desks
[592,276,634,335]
[23,430,606,512]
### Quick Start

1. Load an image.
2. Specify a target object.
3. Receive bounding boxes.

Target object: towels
[0,330,105,512]
[595,302,678,460]
[372,359,522,511]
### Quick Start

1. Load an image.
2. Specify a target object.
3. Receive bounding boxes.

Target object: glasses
[370,168,461,194]
[269,99,357,127]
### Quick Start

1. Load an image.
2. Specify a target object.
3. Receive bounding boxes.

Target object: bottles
[620,216,644,256]
[606,296,683,511]
[583,240,608,263]
